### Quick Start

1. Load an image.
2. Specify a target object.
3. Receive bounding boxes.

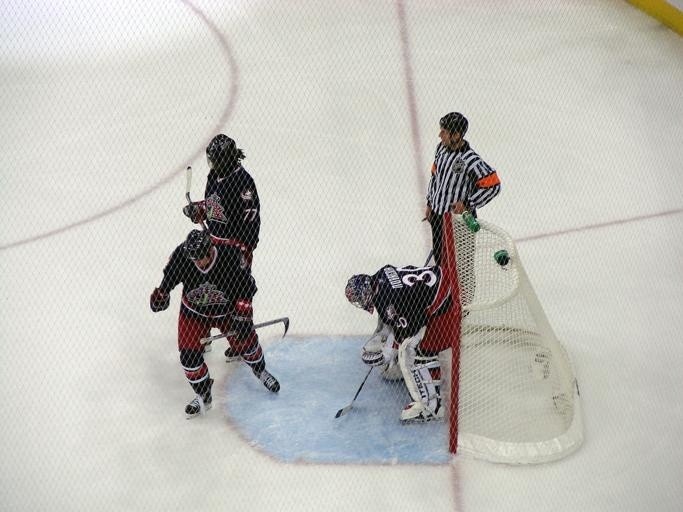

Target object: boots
[185,377,214,416]
[251,361,280,393]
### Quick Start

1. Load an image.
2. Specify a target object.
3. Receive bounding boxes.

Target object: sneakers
[225,347,242,357]
[413,405,447,422]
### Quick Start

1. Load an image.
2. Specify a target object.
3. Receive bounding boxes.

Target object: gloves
[150,287,172,312]
[236,241,253,272]
[230,310,255,341]
[362,351,388,369]
[183,201,210,224]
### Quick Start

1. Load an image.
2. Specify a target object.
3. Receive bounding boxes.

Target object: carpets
[219,332,453,468]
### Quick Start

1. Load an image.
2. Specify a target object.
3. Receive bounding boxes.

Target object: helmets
[206,135,239,175]
[345,273,379,311]
[182,227,212,262]
[439,112,468,140]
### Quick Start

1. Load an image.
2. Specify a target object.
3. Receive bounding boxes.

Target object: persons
[183,133,260,362]
[344,265,457,422]
[149,229,280,419]
[425,112,501,265]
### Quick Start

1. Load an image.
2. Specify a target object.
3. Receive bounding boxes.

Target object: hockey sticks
[200,318,289,345]
[335,366,373,418]
[186,167,207,231]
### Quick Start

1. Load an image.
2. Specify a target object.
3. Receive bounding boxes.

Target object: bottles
[460,209,480,232]
